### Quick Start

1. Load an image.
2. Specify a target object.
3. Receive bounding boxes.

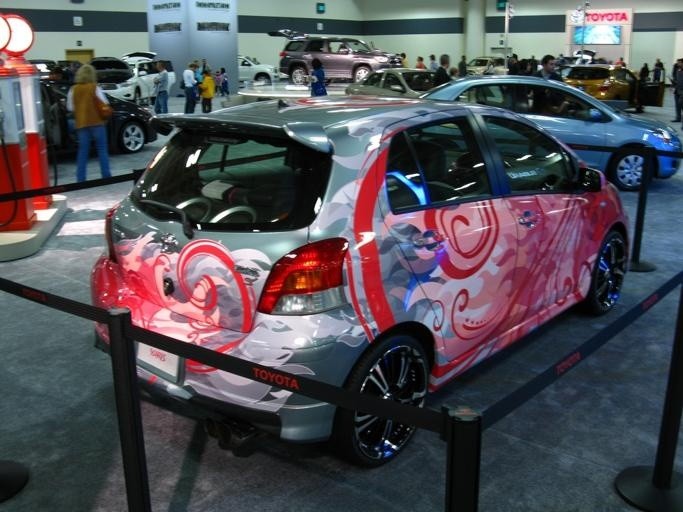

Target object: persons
[181,57,229,115]
[309,59,326,96]
[40,66,64,83]
[616,55,682,131]
[397,51,570,118]
[65,62,114,186]
[151,60,169,116]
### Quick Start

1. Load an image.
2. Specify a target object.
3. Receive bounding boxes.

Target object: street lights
[569,0,591,64]
[503,1,516,69]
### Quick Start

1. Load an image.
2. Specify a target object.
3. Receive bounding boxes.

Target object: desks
[237,86,310,99]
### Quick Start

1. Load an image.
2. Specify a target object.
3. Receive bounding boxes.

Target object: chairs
[401,139,462,200]
[137,63,148,76]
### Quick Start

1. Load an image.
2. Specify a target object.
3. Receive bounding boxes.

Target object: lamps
[1,14,35,55]
[0,12,11,51]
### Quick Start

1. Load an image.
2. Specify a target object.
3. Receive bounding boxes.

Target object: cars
[556,63,665,110]
[36,77,157,157]
[90,92,630,471]
[343,67,457,98]
[418,64,682,192]
[28,59,84,81]
[237,54,279,84]
[535,46,599,73]
[466,55,509,75]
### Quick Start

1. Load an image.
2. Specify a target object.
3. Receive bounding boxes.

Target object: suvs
[267,29,404,85]
[87,51,177,107]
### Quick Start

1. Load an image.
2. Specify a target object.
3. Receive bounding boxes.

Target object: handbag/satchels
[93,97,113,119]
